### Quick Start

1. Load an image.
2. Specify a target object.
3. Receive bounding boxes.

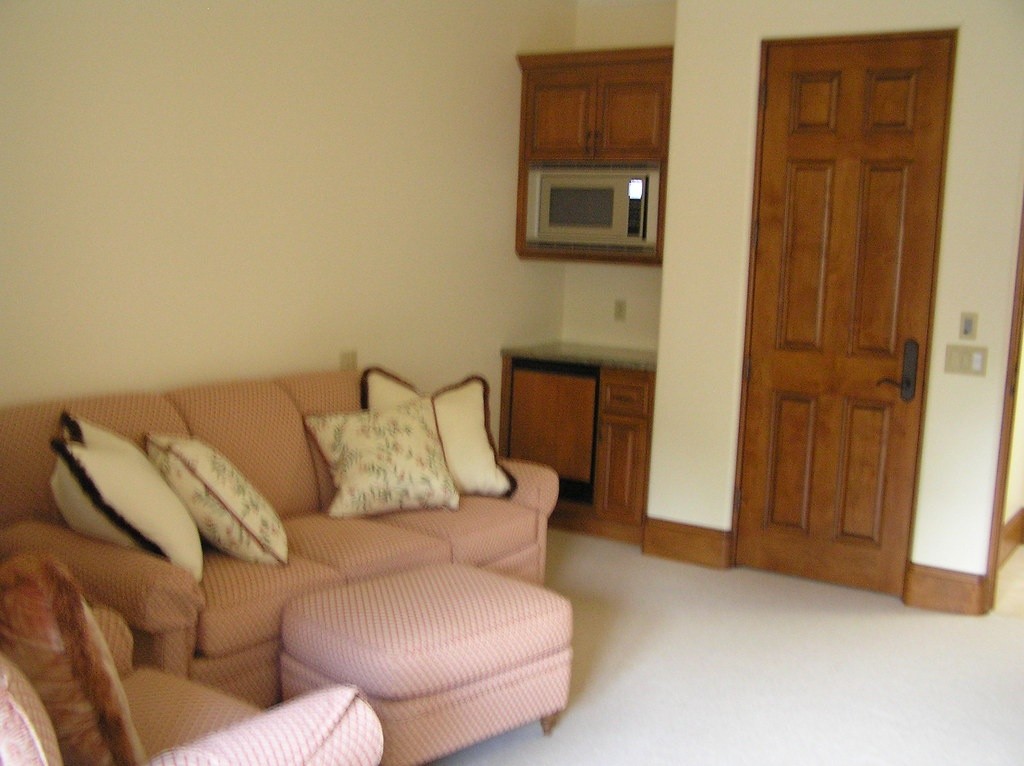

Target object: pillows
[52,413,204,583]
[358,369,516,500]
[303,400,460,517]
[144,430,288,564]
[3,549,144,766]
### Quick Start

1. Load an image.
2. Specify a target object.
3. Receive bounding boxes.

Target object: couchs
[0,365,561,766]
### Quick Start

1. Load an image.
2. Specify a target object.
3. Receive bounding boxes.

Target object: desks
[277,562,573,766]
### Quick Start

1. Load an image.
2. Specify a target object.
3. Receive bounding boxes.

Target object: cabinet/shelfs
[504,362,650,548]
[512,49,671,264]
